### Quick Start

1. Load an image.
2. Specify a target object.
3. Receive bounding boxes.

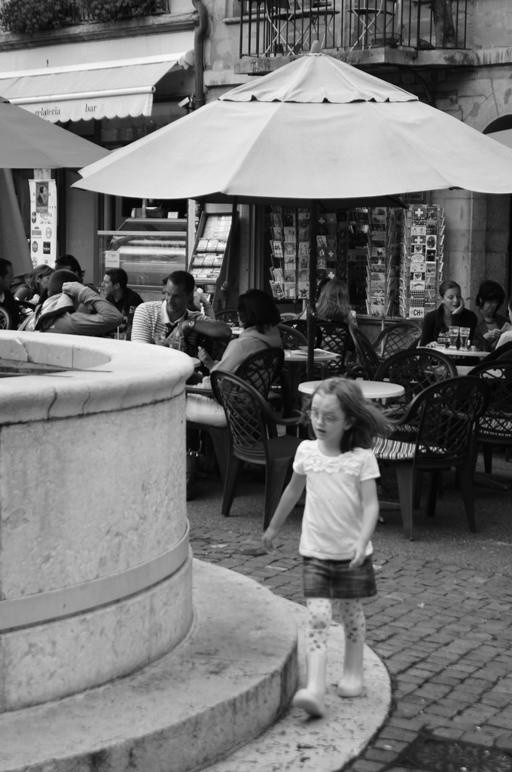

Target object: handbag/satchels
[343,346,379,377]
[220,375,287,409]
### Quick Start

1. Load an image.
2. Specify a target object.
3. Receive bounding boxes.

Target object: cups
[459,327,471,351]
[448,326,460,350]
[115,333,127,341]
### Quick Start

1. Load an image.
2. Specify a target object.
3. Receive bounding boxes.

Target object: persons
[471,280,511,351]
[36,185,47,212]
[197,289,284,477]
[260,377,390,717]
[289,279,362,370]
[469,301,512,438]
[1,255,231,501]
[419,280,477,350]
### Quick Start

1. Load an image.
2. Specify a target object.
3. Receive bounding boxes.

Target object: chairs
[260,0,396,57]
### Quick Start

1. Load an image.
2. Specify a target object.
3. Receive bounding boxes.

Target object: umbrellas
[0,169,33,277]
[0,97,111,169]
[70,39,512,381]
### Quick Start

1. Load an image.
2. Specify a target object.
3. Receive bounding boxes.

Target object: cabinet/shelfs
[270,205,445,319]
[187,211,240,312]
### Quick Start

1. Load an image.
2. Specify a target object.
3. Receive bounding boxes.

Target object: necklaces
[484,320,495,324]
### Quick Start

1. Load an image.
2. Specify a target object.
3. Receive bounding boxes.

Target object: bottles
[127,305,134,337]
[169,321,185,352]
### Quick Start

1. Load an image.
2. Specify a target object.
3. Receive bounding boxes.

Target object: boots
[293,651,327,717]
[337,639,364,698]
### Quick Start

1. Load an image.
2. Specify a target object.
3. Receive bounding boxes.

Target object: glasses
[306,409,337,424]
